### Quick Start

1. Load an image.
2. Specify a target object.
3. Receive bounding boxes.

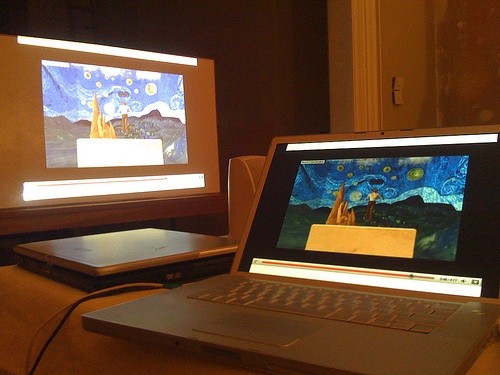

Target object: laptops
[15,227,238,292]
[82,124,500,375]
